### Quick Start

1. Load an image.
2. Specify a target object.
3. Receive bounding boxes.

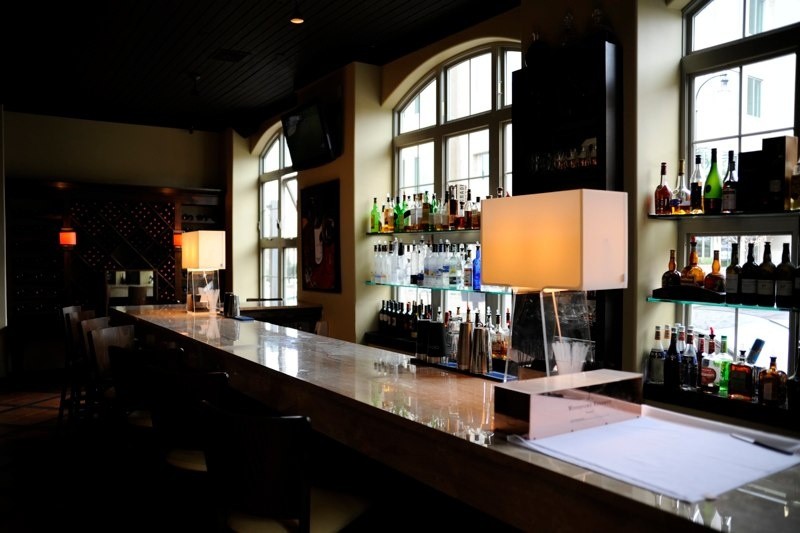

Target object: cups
[225,292,241,318]
[415,319,493,376]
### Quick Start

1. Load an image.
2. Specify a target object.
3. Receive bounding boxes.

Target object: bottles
[662,249,682,286]
[721,151,738,214]
[704,148,721,214]
[370,236,482,290]
[379,299,512,360]
[370,184,503,234]
[537,144,598,172]
[777,243,794,307]
[742,242,759,306]
[654,162,670,215]
[726,243,742,305]
[705,250,726,293]
[670,159,689,215]
[690,154,704,215]
[681,235,705,288]
[759,241,777,307]
[647,322,800,413]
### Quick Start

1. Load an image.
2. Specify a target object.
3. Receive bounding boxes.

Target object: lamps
[478,189,645,443]
[182,230,228,314]
[58,222,77,252]
[173,230,186,252]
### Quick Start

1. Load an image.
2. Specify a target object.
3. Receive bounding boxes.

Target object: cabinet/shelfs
[510,38,621,195]
[367,228,513,363]
[647,210,800,414]
[177,175,223,310]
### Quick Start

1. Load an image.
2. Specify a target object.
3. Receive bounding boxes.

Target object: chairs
[55,305,212,476]
[194,399,311,533]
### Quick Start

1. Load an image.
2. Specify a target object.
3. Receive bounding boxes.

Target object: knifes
[730,431,794,455]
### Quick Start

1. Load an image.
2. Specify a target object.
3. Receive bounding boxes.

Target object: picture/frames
[300,177,343,295]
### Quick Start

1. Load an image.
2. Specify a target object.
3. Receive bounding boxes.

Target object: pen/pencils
[730,432,794,456]
